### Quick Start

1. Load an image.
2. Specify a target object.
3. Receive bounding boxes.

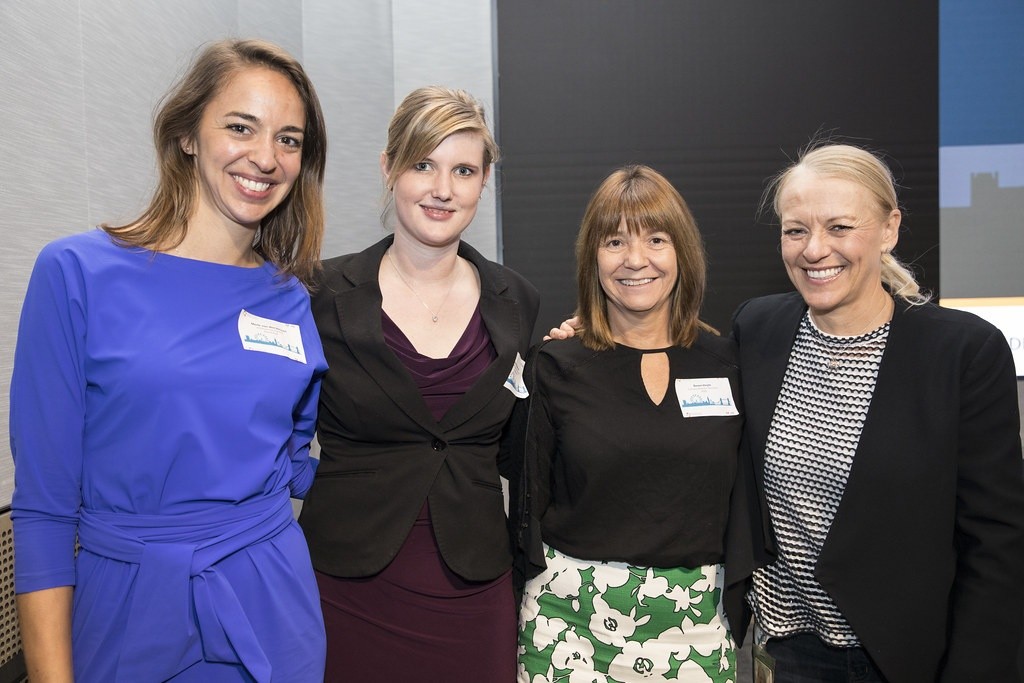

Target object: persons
[543,146,1023,682]
[9,40,329,683]
[294,84,543,683]
[507,165,745,681]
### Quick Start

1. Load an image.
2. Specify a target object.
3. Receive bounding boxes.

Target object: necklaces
[810,289,887,370]
[388,245,460,322]
